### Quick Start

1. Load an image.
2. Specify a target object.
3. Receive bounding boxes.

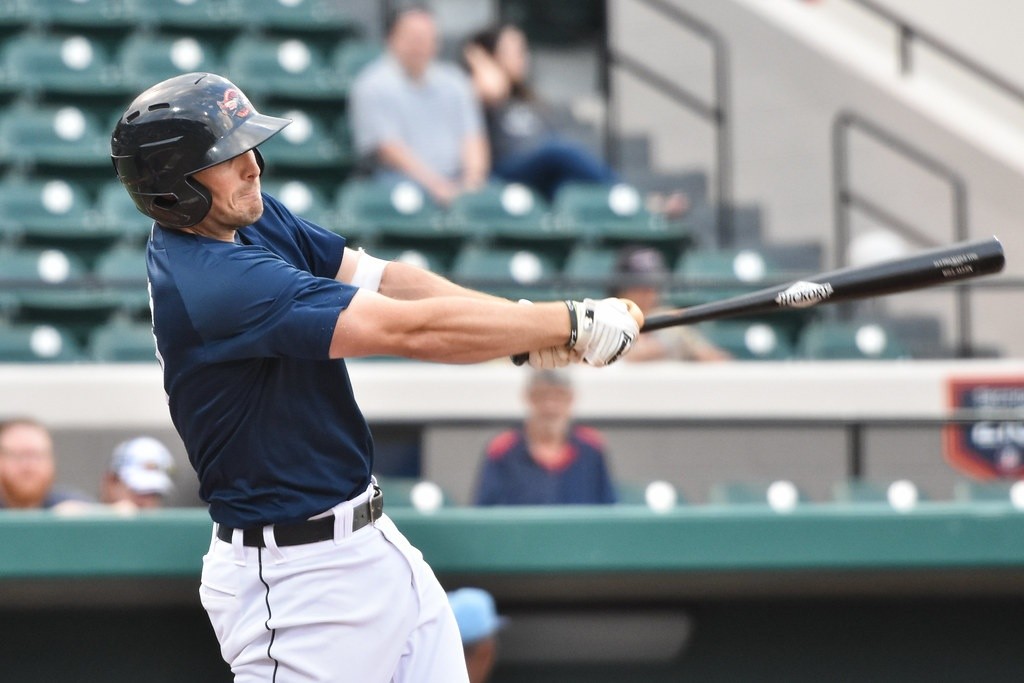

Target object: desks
[0,499,1023,604]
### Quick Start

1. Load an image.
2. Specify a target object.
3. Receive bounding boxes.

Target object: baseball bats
[507,234,1007,369]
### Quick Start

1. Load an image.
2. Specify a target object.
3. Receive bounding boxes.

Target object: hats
[110,438,174,498]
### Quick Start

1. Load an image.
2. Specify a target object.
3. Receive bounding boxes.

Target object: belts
[217,486,384,547]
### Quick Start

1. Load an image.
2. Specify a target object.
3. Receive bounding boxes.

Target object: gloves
[510,299,572,370]
[565,297,640,368]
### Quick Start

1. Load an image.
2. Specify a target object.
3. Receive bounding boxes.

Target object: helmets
[110,72,293,227]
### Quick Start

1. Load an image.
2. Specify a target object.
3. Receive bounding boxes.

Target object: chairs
[0,0,948,362]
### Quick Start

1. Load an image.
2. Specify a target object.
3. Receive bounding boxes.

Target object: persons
[110,71,647,683]
[602,245,734,362]
[0,418,178,516]
[446,586,511,682]
[467,367,617,509]
[347,0,689,219]
